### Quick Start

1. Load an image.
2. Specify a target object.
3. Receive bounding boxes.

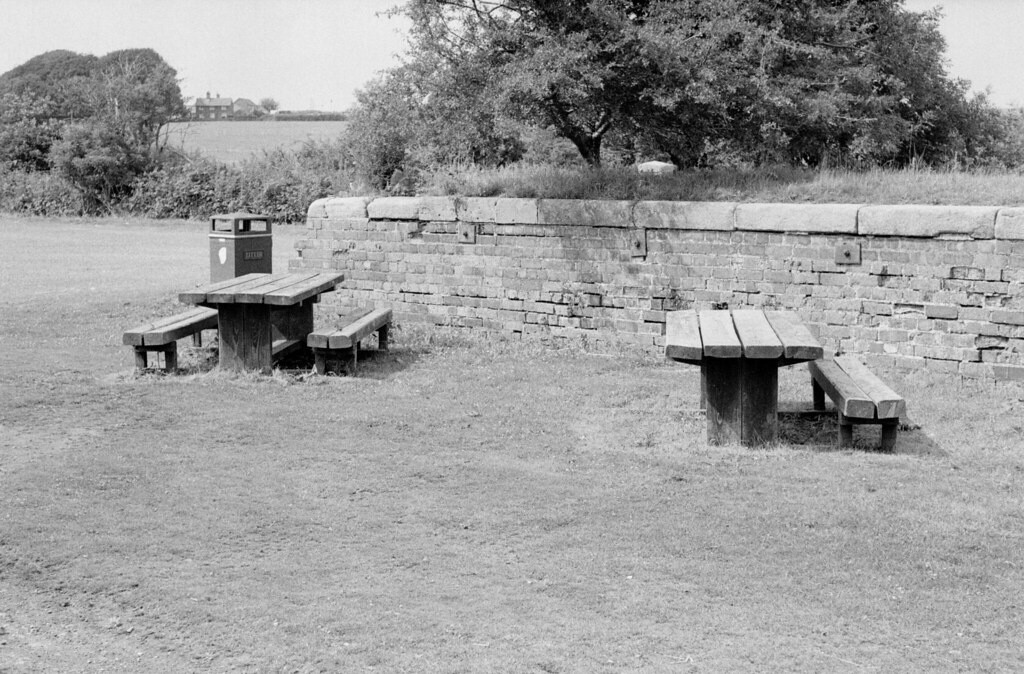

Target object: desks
[178,272,346,377]
[665,309,824,447]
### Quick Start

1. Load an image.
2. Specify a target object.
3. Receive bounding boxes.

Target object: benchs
[122,306,218,376]
[307,306,392,377]
[808,356,906,454]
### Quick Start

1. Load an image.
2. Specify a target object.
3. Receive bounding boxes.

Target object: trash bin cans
[209,211,274,283]
